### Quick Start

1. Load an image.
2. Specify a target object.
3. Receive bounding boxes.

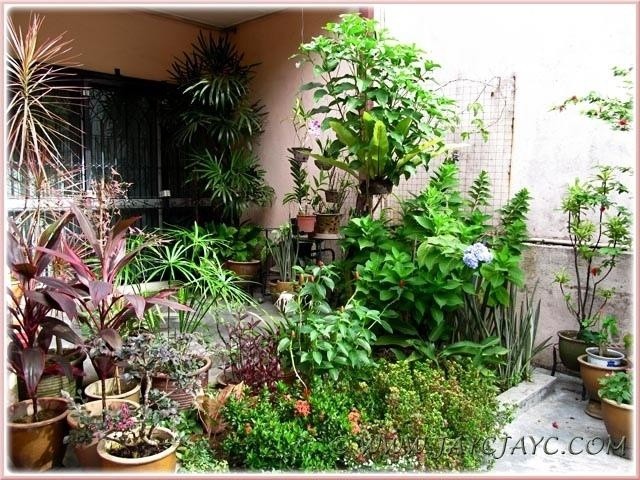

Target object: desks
[297,234,345,269]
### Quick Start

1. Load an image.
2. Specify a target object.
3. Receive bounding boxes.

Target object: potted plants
[172,32,273,287]
[285,146,358,234]
[266,228,300,302]
[5,217,285,472]
[552,165,633,462]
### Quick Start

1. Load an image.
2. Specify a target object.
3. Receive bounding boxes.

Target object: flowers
[292,96,323,146]
